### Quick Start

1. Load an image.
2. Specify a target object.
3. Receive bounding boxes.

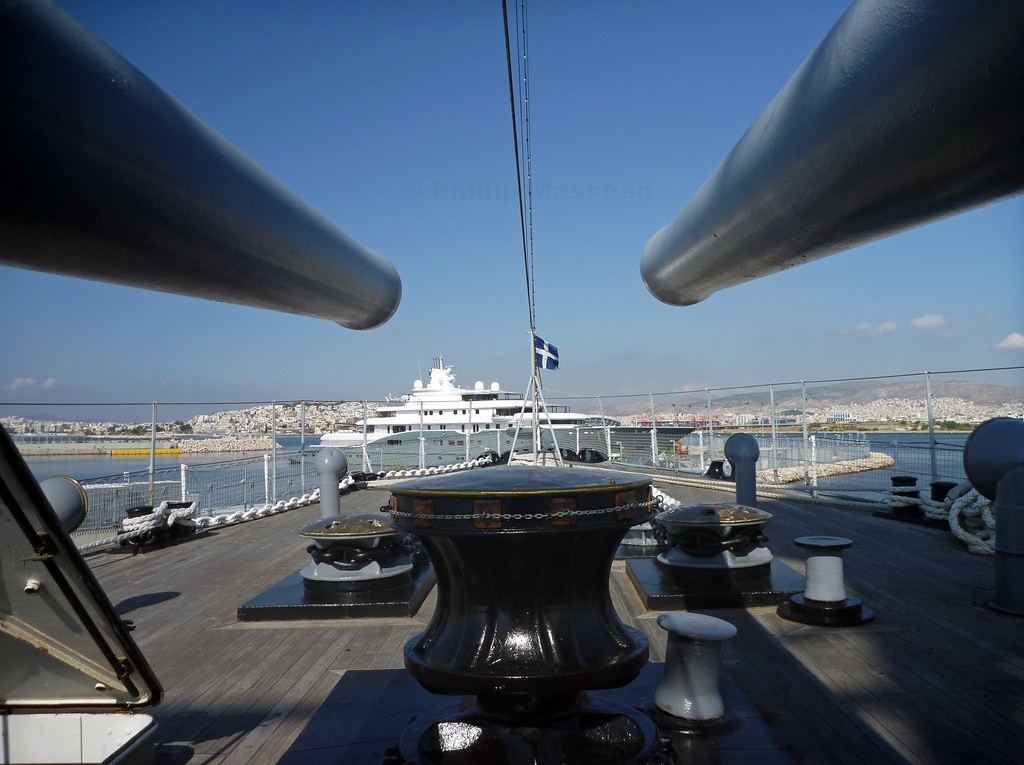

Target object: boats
[287,355,699,468]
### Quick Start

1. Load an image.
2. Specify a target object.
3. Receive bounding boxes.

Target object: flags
[533,333,559,370]
